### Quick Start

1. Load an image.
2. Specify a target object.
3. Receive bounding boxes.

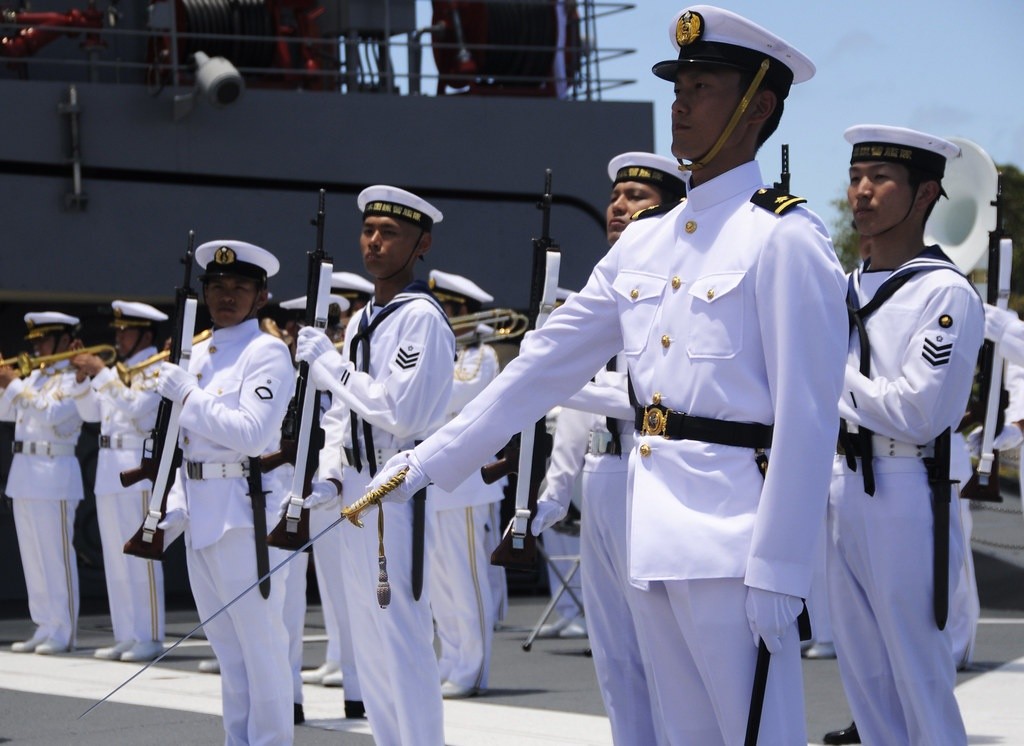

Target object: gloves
[981,304,1019,344]
[157,508,189,554]
[964,418,1022,460]
[278,481,339,520]
[500,497,567,541]
[356,450,431,518]
[156,361,197,406]
[744,585,804,656]
[296,323,337,369]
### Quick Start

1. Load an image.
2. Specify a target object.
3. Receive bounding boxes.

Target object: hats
[24,312,79,340]
[357,184,443,233]
[845,126,960,178]
[279,293,350,333]
[326,272,376,301]
[429,268,494,307]
[108,300,169,329]
[652,6,816,102]
[195,240,280,289]
[607,152,693,196]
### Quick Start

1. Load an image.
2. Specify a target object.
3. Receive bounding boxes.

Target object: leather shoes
[824,721,860,744]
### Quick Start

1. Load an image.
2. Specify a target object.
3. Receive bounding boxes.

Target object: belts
[9,404,929,480]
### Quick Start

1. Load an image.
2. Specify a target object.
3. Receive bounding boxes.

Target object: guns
[773,140,794,195]
[258,186,337,553]
[119,228,201,561]
[954,169,1014,505]
[478,168,563,569]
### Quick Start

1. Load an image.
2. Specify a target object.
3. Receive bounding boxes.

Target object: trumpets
[333,306,530,355]
[0,344,117,379]
[260,316,293,346]
[116,329,213,389]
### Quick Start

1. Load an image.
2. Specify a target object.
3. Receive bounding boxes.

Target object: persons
[0,154,1024,746]
[819,124,985,746]
[367,6,851,746]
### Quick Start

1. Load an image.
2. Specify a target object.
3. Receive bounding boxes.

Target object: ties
[833,271,918,497]
[606,353,617,459]
[349,302,402,478]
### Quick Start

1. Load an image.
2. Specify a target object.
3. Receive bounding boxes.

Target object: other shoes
[10,606,840,701]
[345,701,364,717]
[294,703,305,724]
